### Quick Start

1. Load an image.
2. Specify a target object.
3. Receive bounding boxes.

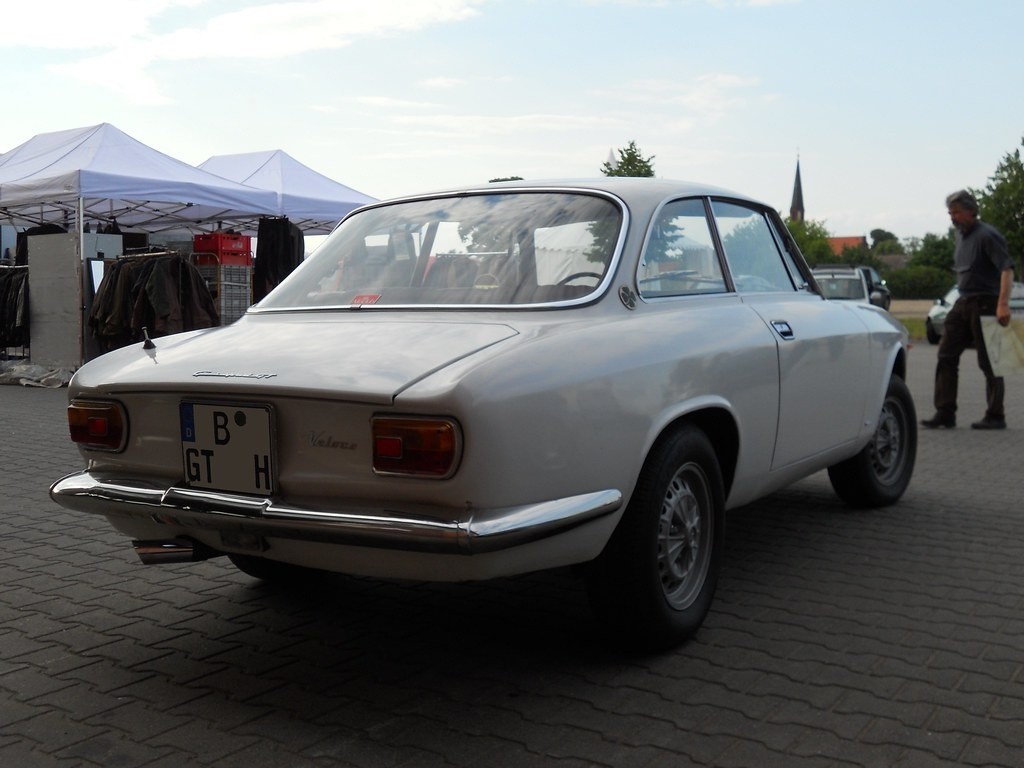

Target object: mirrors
[87,257,104,296]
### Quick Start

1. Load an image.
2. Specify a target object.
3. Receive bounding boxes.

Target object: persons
[919,189,1014,432]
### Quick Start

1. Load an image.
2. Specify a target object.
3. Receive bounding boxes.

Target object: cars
[810,264,870,305]
[48,171,919,651]
[925,280,1024,345]
[732,274,776,292]
[859,266,892,310]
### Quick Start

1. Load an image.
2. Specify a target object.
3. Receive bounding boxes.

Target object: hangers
[0,265,28,275]
[119,251,183,265]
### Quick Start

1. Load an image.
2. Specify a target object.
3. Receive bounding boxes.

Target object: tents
[0,122,367,368]
[192,149,386,236]
[300,223,715,295]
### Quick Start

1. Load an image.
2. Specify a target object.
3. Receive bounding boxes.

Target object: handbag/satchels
[981,314,1024,376]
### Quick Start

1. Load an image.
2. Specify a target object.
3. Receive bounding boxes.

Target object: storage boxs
[194,233,251,326]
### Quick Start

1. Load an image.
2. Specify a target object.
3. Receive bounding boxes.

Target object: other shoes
[970,409,1008,430]
[921,412,957,429]
